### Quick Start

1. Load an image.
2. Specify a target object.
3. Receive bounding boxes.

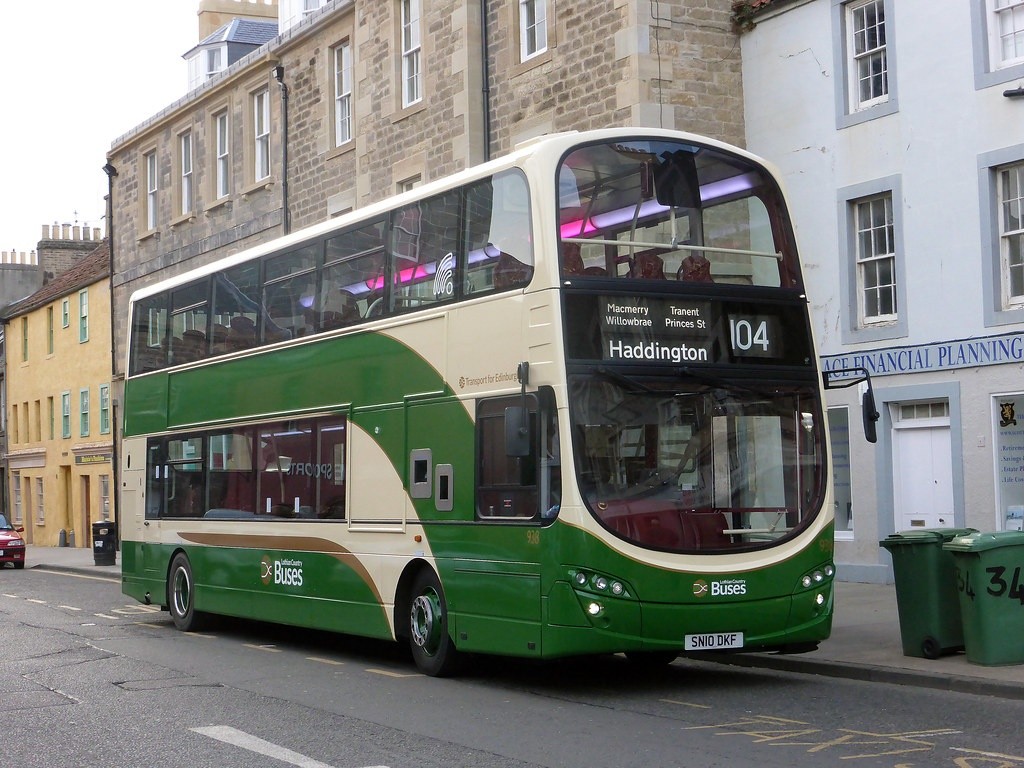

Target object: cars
[0,512,27,570]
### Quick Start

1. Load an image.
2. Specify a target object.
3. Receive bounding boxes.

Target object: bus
[117,125,882,681]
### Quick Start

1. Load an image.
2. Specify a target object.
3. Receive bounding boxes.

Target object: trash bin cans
[942,530,1024,667]
[91,520,117,566]
[879,527,980,659]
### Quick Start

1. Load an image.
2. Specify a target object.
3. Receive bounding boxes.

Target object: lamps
[1004,87,1024,98]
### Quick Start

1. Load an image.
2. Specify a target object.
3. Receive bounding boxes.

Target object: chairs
[173,466,332,517]
[141,240,717,370]
